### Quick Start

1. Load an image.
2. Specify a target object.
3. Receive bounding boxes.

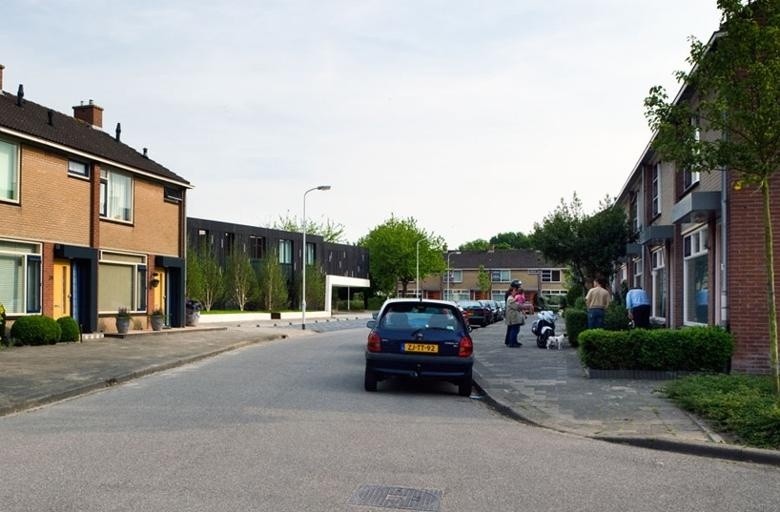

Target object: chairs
[387,312,453,329]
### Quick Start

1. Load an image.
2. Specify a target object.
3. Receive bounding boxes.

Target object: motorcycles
[532,311,554,348]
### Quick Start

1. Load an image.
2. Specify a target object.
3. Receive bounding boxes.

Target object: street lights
[447,251,461,301]
[415,235,431,297]
[302,185,331,330]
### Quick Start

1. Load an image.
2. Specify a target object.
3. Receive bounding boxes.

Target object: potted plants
[115,306,133,334]
[147,307,173,331]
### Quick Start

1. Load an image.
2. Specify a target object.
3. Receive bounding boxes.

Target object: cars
[364,298,475,397]
[455,299,506,327]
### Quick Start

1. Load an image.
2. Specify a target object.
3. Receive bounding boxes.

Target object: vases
[186,309,201,326]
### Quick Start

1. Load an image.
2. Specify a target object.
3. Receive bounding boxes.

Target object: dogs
[545,334,569,350]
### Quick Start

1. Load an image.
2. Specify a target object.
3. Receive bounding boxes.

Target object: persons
[505,287,531,347]
[510,287,527,319]
[626,286,652,329]
[585,275,611,328]
[504,279,522,345]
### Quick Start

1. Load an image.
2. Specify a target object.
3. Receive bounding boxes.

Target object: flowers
[185,299,204,311]
